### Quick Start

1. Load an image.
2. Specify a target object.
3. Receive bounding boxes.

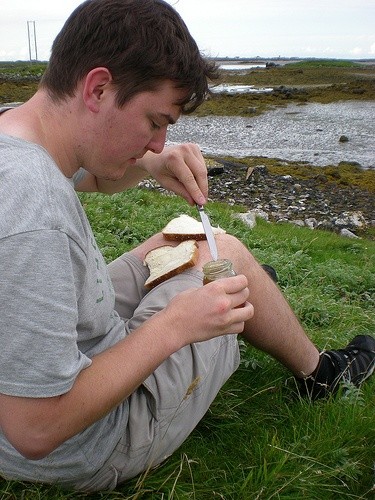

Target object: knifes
[195,201,218,262]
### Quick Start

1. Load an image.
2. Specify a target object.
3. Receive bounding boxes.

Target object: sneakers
[260,265,278,285]
[307,335,374,403]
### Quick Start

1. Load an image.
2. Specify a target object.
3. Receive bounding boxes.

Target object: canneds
[202,257,246,309]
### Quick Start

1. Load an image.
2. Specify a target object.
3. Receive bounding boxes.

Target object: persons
[1,0,375,492]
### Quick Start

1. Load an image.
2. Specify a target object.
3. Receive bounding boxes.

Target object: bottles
[203,259,245,309]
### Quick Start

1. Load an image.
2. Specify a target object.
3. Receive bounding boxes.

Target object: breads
[143,214,225,289]
